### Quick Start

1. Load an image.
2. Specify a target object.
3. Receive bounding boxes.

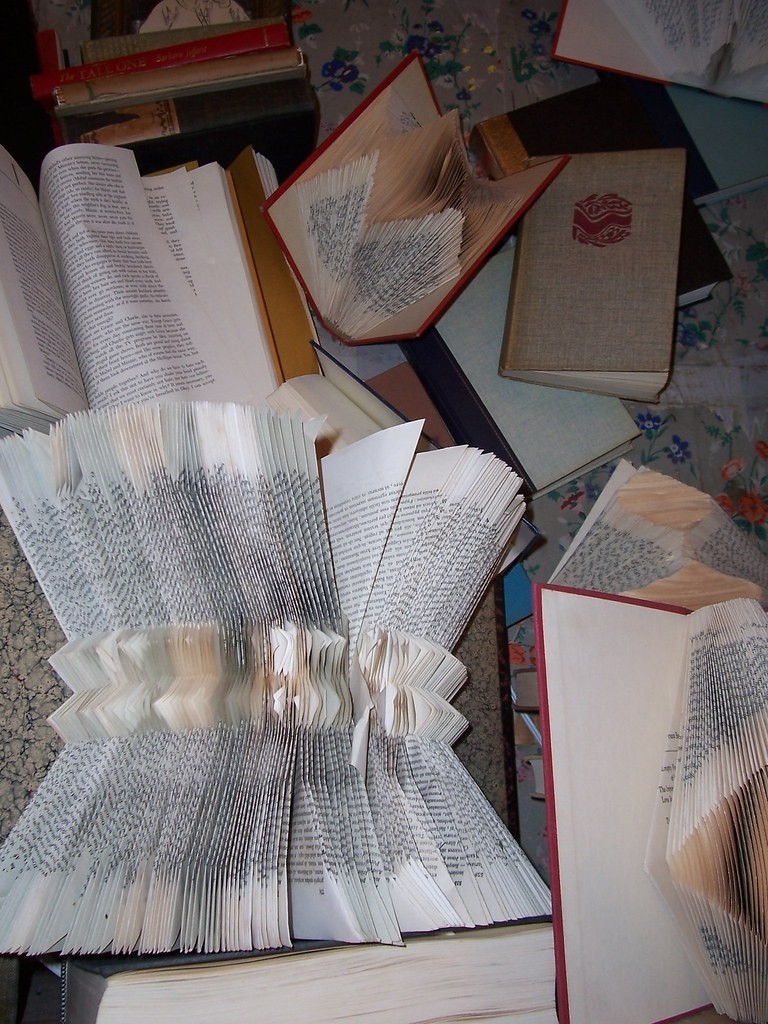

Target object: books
[0,0,768,1022]
